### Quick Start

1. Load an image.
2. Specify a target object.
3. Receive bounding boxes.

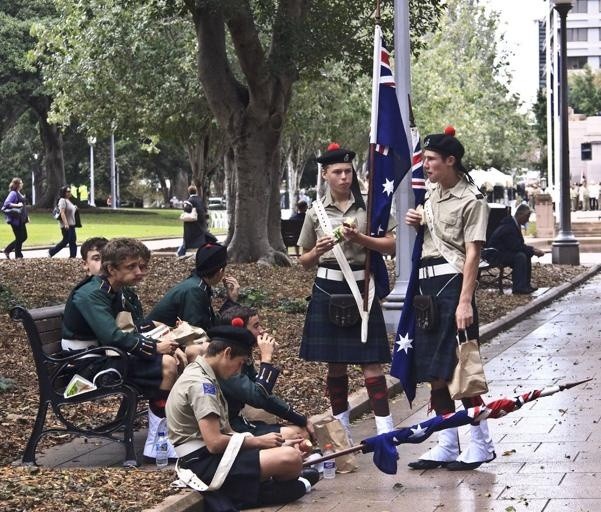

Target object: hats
[207,318,255,347]
[317,143,355,163]
[196,243,227,271]
[424,127,464,158]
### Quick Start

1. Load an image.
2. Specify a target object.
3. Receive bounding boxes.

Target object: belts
[60,339,97,350]
[418,263,459,280]
[317,267,366,282]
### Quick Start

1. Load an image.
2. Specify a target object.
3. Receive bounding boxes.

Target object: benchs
[280,218,305,258]
[10,304,148,467]
[477,247,512,294]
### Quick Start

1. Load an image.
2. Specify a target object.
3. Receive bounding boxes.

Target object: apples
[298,439,312,451]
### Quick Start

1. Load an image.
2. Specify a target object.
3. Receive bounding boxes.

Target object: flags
[391,127,425,409]
[371,40,410,301]
[362,388,542,475]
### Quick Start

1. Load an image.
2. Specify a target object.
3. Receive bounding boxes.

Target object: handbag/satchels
[449,341,488,400]
[413,295,439,330]
[180,208,197,222]
[52,207,61,220]
[205,232,217,242]
[329,294,356,327]
[1,205,22,217]
[314,416,358,473]
[75,209,81,227]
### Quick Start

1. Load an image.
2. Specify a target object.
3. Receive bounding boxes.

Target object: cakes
[343,215,359,231]
[163,318,202,340]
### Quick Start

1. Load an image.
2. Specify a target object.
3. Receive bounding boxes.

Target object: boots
[408,425,459,469]
[142,405,176,462]
[449,422,495,471]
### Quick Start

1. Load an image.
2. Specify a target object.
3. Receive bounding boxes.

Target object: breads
[416,202,427,225]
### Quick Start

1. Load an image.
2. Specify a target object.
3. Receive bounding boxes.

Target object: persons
[48,185,77,258]
[286,201,308,259]
[62,236,210,464]
[220,306,316,443]
[487,204,542,293]
[2,178,27,258]
[165,318,318,510]
[177,187,218,254]
[106,194,112,205]
[405,128,489,468]
[297,142,396,460]
[143,242,239,333]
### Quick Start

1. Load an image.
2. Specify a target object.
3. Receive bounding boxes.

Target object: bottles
[323,444,335,478]
[156,431,168,466]
